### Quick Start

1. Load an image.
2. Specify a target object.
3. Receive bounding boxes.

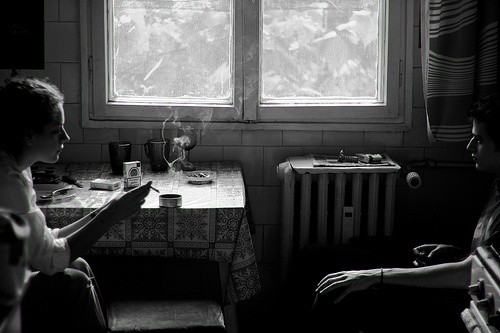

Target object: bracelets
[91,212,95,218]
[381,268,384,282]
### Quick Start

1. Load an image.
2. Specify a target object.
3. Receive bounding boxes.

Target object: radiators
[274,155,402,289]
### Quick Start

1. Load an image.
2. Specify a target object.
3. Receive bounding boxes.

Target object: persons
[316,96,500,333]
[0,77,153,333]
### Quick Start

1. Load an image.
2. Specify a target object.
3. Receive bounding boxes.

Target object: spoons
[40,185,73,197]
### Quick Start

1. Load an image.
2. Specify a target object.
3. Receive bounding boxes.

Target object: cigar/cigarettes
[150,186,159,192]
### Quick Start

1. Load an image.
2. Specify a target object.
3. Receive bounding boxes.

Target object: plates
[33,184,78,200]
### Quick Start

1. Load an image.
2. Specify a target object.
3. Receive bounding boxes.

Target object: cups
[109,141,131,174]
[144,138,170,172]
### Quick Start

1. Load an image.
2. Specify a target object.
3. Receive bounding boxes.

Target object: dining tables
[31,163,261,333]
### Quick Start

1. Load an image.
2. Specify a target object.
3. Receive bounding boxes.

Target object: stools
[105,297,226,333]
